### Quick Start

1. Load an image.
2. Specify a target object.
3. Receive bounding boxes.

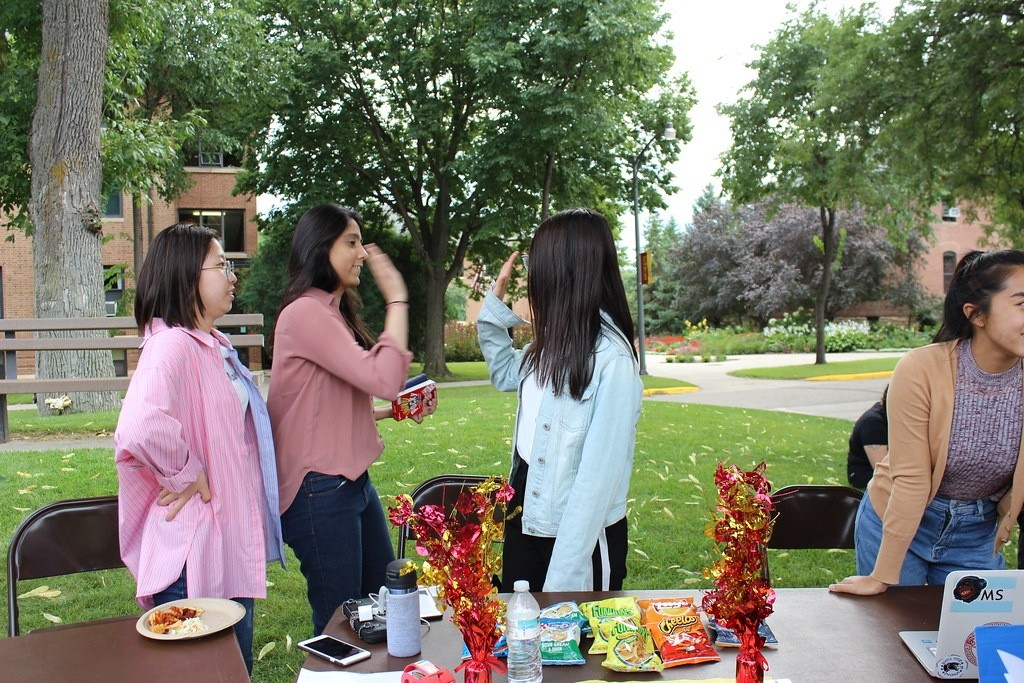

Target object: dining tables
[0,618,251,683]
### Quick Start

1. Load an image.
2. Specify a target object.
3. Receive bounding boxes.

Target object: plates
[136,597,246,640]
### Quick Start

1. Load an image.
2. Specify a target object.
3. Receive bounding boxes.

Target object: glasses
[520,252,530,271]
[200,258,235,283]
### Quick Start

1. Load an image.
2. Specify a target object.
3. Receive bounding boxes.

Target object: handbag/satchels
[342,594,390,643]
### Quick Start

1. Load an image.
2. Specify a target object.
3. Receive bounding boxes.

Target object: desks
[296,584,977,683]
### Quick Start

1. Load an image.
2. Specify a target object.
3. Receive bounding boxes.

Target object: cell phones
[297,634,372,668]
[418,587,443,622]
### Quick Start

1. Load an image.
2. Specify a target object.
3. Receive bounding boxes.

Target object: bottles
[506,580,543,683]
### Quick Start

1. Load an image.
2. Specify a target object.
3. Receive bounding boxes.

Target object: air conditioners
[105,300,118,316]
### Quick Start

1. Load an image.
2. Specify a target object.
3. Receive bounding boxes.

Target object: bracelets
[385,300,409,310]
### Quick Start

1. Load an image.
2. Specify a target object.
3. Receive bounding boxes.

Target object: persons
[829,248,1024,596]
[267,204,438,637]
[478,210,645,592]
[847,385,888,488]
[115,222,287,675]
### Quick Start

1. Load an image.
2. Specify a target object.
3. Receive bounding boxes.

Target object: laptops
[899,570,1024,679]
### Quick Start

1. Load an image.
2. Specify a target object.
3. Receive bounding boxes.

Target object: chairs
[398,474,505,560]
[0,496,141,638]
[760,485,865,579]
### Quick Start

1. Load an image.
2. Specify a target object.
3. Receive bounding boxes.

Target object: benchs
[0,313,264,444]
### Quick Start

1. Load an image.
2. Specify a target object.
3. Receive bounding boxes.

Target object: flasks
[386,558,421,657]
